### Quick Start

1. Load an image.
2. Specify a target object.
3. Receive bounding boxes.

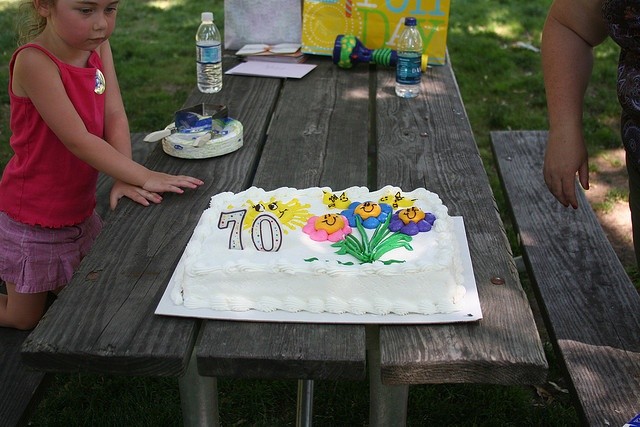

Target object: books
[242,43,308,63]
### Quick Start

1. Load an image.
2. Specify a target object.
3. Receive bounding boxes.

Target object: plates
[153,213,483,323]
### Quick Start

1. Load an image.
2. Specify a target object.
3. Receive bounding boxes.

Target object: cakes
[171,185,468,316]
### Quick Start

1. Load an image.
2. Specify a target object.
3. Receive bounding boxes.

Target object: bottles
[333,35,428,72]
[196,12,223,93]
[395,17,423,99]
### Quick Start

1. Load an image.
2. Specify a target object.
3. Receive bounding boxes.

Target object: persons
[543,0,639,271]
[0,0,203,330]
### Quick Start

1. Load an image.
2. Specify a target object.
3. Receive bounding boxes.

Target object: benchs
[485,125,640,427]
[0,129,164,426]
